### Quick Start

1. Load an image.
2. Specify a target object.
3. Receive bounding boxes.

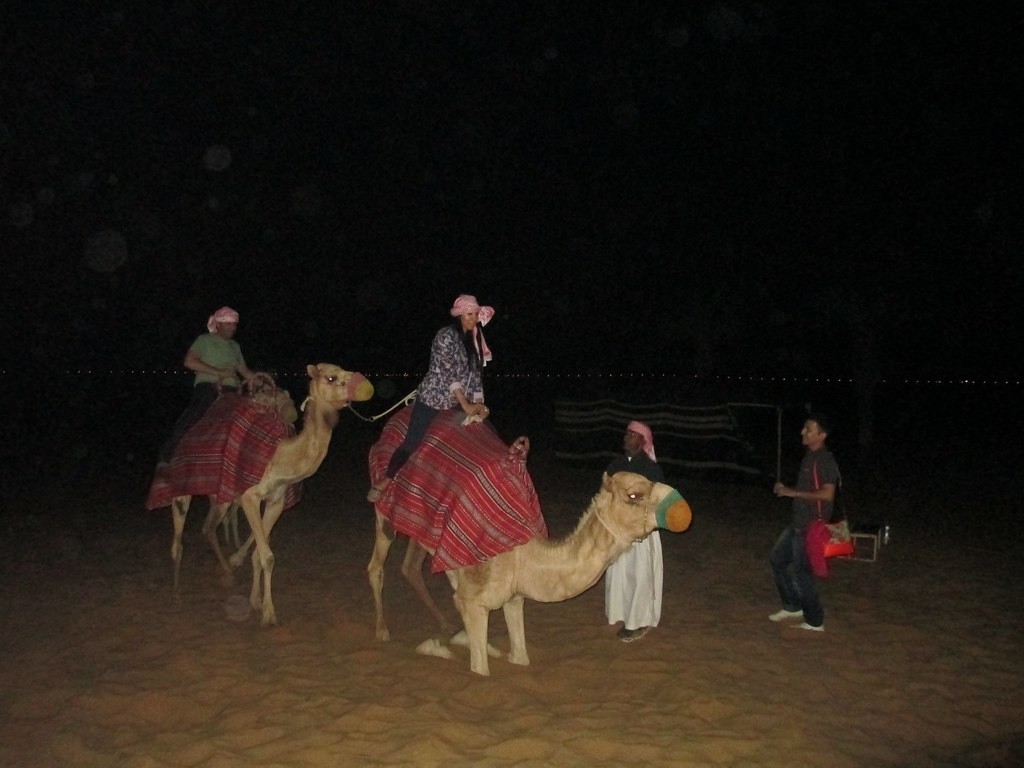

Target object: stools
[835,520,883,562]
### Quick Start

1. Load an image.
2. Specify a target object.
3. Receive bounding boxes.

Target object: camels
[366,400,693,676]
[143,362,375,630]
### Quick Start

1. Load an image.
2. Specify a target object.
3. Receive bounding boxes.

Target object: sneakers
[767,609,804,623]
[790,622,825,633]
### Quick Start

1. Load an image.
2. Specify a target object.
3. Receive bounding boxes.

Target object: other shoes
[367,485,383,503]
[157,465,175,479]
[617,626,653,643]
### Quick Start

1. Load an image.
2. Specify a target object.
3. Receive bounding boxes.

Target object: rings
[485,407,488,412]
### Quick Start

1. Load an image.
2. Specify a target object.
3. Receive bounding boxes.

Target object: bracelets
[258,380,265,388]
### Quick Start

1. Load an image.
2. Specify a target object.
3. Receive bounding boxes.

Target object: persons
[603,419,664,644]
[367,293,495,504]
[769,412,841,632]
[170,305,271,451]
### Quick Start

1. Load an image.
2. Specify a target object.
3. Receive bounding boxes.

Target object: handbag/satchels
[804,518,855,578]
